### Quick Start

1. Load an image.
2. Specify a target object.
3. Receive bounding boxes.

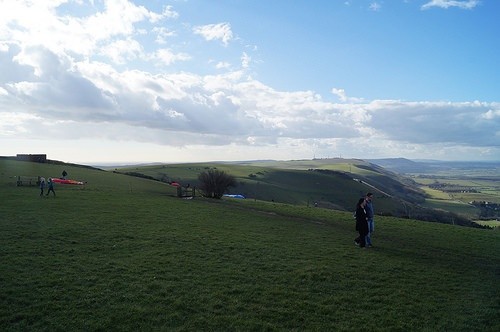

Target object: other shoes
[354,240,373,249]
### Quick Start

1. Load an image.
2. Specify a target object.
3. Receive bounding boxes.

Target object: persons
[46,178,55,196]
[364,192,376,248]
[39,176,46,197]
[353,197,370,250]
